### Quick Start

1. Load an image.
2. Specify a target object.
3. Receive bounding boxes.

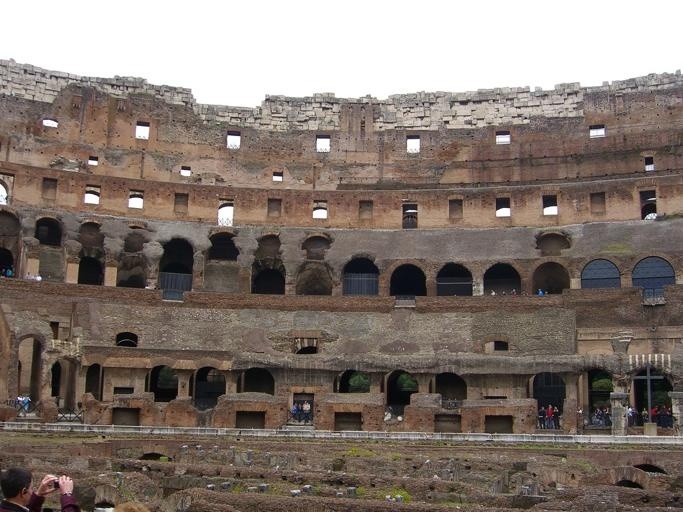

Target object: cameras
[53,480,60,488]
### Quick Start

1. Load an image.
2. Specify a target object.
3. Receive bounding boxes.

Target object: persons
[0,467,82,512]
[536,404,561,429]
[291,400,311,423]
[0,264,43,282]
[627,405,672,427]
[577,403,611,425]
[3,393,31,413]
[487,287,548,296]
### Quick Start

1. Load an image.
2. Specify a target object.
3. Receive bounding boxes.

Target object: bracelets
[62,492,73,497]
